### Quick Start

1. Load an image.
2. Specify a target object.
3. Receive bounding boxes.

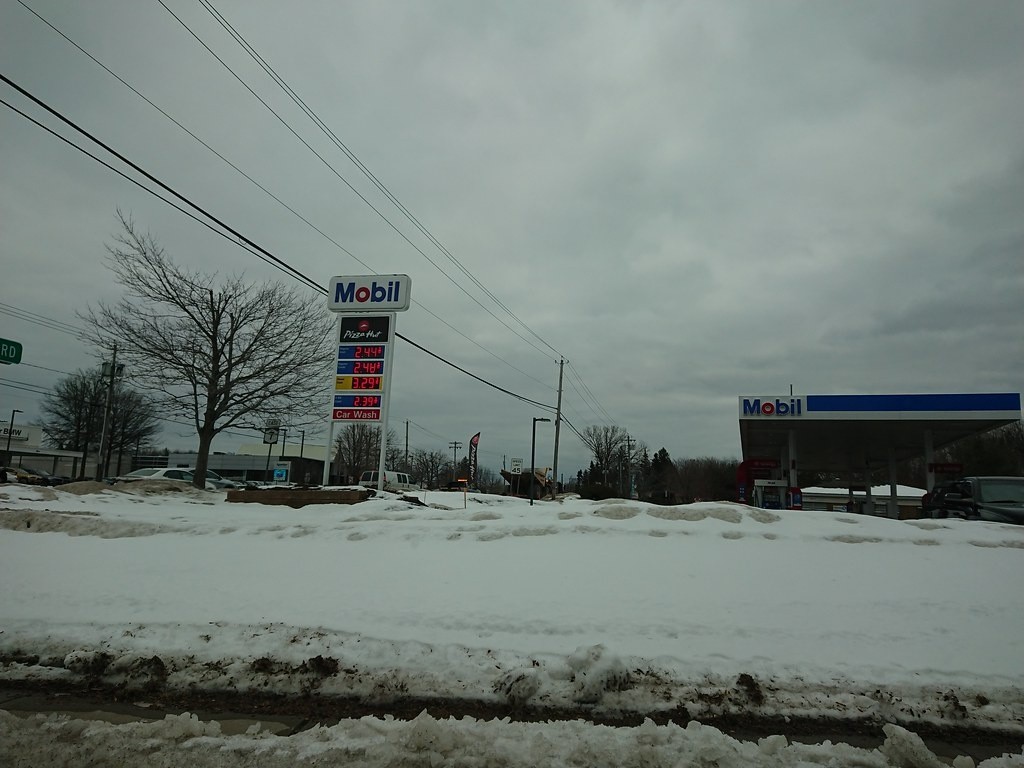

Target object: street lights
[529,417,551,507]
[279,429,288,461]
[297,429,305,485]
[2,410,23,483]
[334,440,343,475]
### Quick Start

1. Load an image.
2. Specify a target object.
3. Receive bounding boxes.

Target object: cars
[103,467,219,493]
[179,467,236,491]
[19,467,64,487]
[0,467,43,485]
[439,479,480,493]
[232,480,323,490]
[920,475,1024,526]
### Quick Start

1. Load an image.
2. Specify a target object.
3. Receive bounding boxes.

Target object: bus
[359,471,420,491]
